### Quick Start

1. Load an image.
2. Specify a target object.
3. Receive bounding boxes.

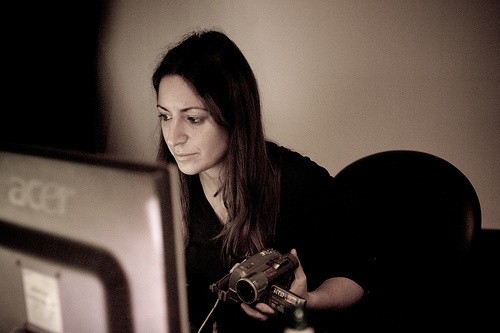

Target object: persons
[151,31,383,333]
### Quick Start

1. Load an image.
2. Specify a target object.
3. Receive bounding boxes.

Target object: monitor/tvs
[0,141,190,333]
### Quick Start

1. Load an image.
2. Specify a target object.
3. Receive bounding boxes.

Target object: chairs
[334,151,481,333]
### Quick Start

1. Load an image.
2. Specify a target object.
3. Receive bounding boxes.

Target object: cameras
[209,248,307,320]
